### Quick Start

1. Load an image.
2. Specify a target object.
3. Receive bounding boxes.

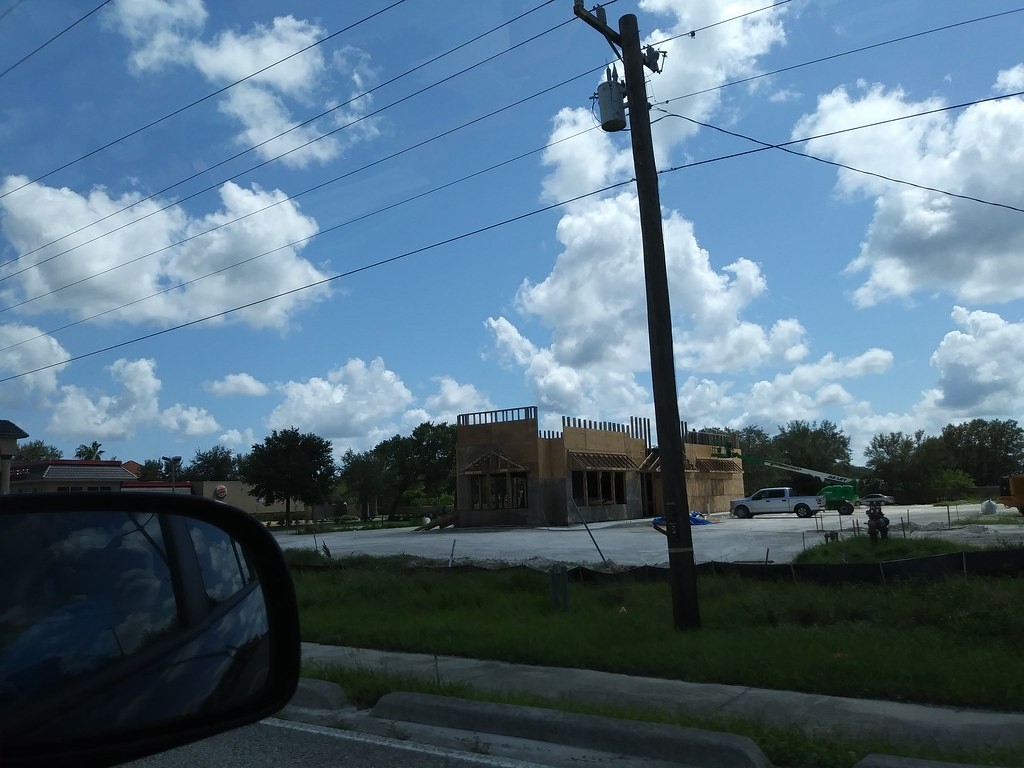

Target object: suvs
[815,478,856,515]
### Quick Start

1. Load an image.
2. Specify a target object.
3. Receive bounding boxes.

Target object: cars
[852,493,896,506]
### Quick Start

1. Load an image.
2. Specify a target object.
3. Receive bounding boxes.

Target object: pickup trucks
[730,487,826,518]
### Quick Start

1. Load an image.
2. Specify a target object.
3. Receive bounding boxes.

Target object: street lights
[162,456,182,494]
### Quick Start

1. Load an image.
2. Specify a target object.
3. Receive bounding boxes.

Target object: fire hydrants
[865,502,890,545]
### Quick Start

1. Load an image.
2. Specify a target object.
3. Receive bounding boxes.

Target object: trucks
[999,474,1024,518]
